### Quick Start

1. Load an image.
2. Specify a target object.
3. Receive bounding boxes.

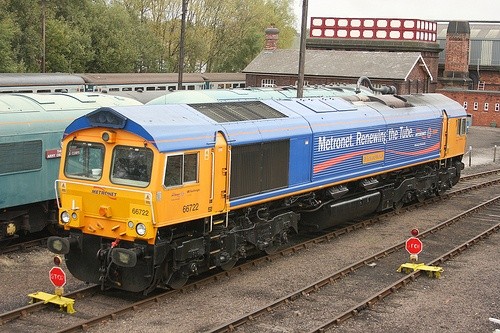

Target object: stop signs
[405,237,423,255]
[49,267,66,287]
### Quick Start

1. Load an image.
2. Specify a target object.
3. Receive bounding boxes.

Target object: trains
[1,73,247,94]
[47,76,467,295]
[0,83,373,245]
